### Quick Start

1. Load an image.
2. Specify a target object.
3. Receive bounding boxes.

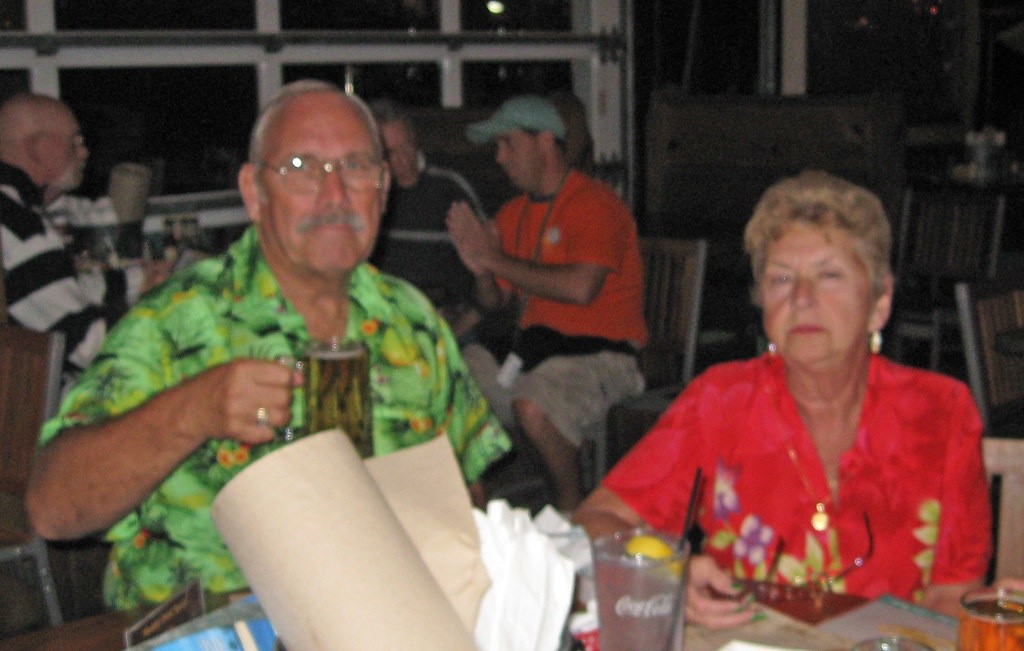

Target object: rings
[257,407,267,424]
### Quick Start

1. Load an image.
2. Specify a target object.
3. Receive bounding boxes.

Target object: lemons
[627,539,683,574]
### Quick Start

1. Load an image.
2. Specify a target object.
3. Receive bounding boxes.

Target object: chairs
[577,169,1024,575]
[0,324,63,626]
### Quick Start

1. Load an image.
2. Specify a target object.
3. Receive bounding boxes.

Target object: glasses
[256,149,385,193]
[751,512,874,606]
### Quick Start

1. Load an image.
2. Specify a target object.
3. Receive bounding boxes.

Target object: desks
[1,575,871,650]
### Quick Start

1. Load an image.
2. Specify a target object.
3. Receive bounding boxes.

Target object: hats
[465,95,566,143]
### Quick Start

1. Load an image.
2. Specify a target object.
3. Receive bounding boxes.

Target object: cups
[164,217,201,263]
[277,342,375,460]
[593,531,690,651]
[957,588,1024,651]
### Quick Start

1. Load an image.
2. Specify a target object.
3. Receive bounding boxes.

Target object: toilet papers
[211,424,492,651]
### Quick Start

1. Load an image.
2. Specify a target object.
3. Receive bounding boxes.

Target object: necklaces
[785,444,841,530]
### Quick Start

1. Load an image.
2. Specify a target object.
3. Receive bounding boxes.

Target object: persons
[25,80,514,614]
[572,169,1024,630]
[447,96,646,508]
[367,96,497,351]
[0,91,173,381]
